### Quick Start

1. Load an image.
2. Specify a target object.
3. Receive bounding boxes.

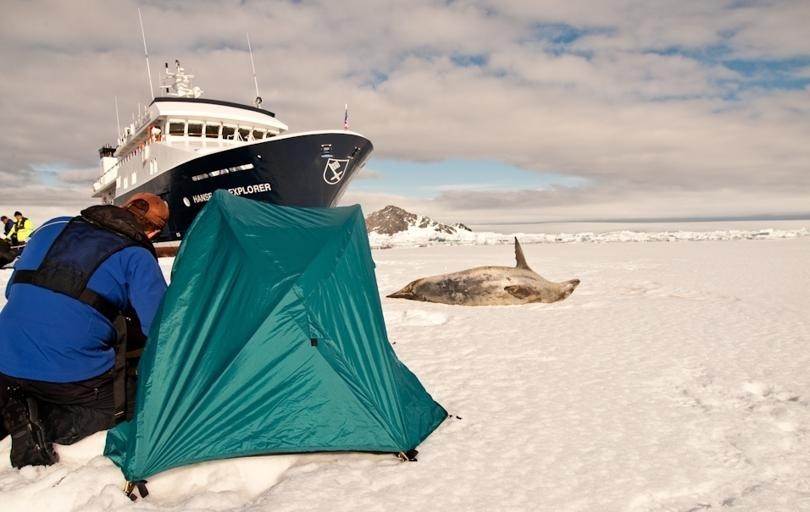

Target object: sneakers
[2,382,59,468]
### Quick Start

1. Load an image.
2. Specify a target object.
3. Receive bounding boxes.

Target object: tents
[101,189,443,489]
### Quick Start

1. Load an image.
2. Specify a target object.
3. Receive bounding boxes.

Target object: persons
[0,191,170,470]
[0,211,34,248]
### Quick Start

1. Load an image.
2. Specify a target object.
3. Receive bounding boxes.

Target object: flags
[344,104,350,129]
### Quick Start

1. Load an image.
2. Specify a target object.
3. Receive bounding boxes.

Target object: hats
[119,192,169,228]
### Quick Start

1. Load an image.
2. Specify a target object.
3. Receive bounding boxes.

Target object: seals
[386,236,581,305]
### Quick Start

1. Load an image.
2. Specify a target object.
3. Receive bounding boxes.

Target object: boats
[90,7,374,255]
